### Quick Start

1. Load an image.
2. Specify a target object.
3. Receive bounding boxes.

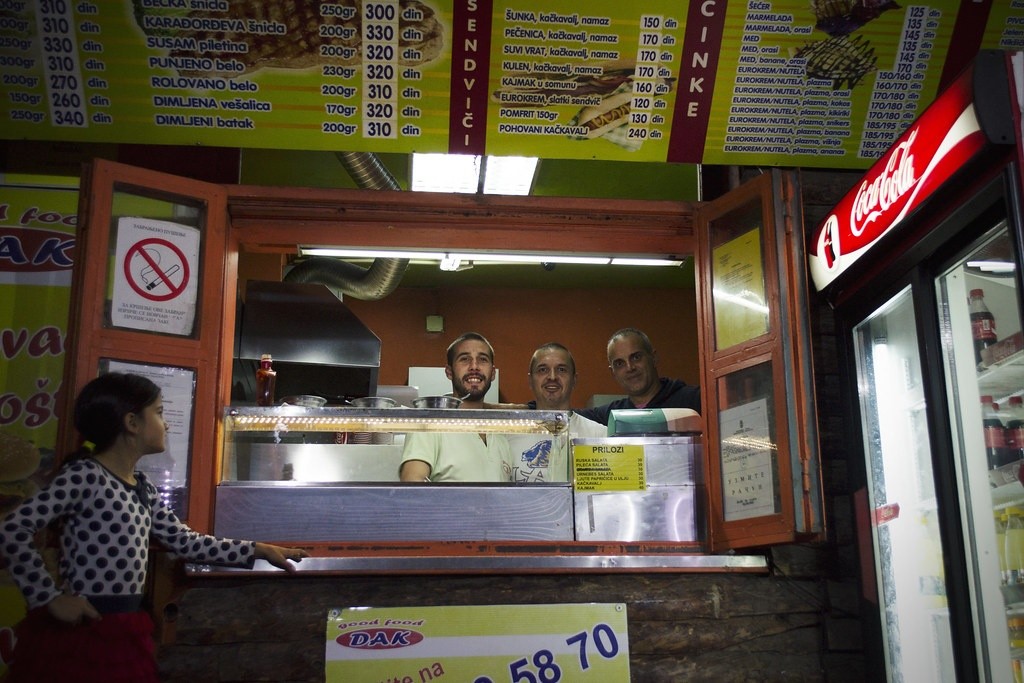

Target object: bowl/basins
[412,395,464,409]
[349,397,397,408]
[279,395,327,408]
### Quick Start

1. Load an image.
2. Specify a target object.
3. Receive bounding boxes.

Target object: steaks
[169,0,444,82]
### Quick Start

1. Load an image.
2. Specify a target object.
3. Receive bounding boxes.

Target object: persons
[528,329,700,425]
[398,332,512,482]
[509,343,607,483]
[0,373,310,683]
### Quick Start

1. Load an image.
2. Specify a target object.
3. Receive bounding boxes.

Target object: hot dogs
[577,94,634,139]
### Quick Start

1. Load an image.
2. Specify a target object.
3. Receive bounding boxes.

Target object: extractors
[230,278,382,407]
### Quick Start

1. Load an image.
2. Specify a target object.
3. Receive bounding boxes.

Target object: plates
[352,432,392,444]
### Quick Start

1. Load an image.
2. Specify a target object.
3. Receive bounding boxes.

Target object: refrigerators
[807,48,1024,683]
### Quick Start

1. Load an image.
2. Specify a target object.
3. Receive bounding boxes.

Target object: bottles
[992,402,1007,432]
[970,289,995,371]
[994,507,1024,585]
[1006,396,1024,462]
[980,395,1006,487]
[256,354,276,407]
[1006,608,1024,683]
